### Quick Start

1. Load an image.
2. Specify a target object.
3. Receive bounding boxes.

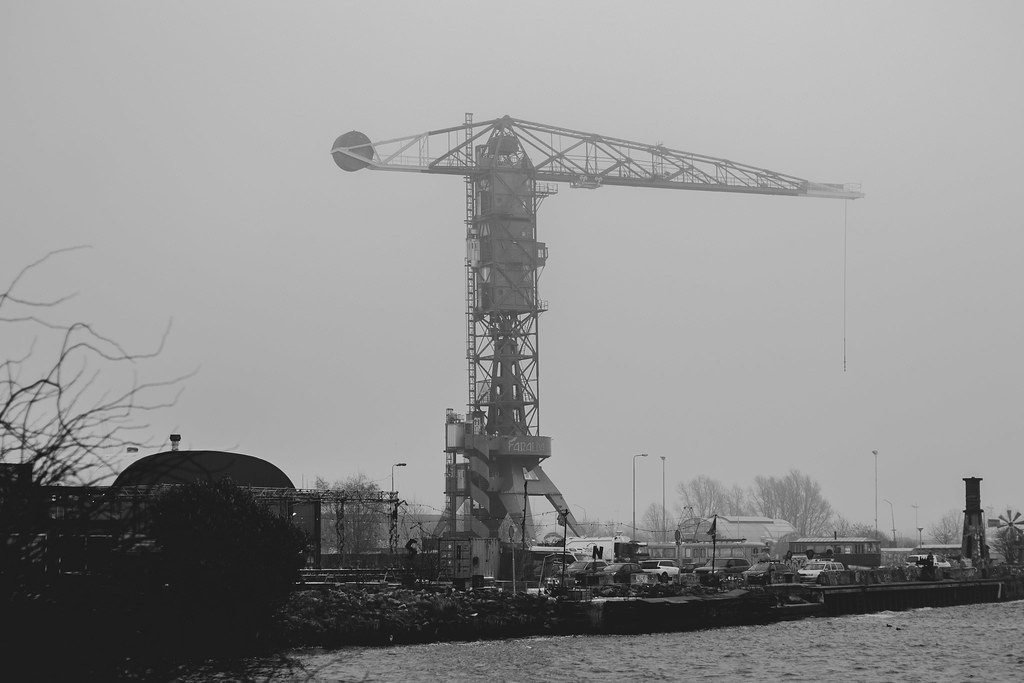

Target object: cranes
[331,114,866,554]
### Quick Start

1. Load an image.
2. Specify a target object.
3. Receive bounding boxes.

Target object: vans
[797,560,844,584]
[905,554,951,567]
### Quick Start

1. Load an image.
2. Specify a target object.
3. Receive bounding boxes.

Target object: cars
[738,563,793,588]
[602,562,640,583]
[566,560,608,585]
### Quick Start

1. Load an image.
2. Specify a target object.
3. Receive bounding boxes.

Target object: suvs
[693,557,749,587]
[641,560,680,584]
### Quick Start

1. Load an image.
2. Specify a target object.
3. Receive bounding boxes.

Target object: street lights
[391,463,407,552]
[632,454,648,542]
[873,450,878,540]
[660,455,666,541]
